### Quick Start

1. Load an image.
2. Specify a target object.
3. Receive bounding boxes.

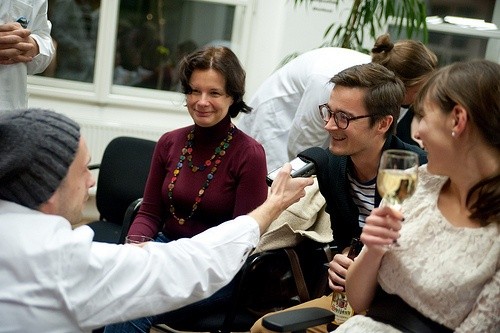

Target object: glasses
[319,104,388,129]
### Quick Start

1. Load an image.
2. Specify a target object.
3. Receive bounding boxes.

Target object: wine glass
[372,149,419,252]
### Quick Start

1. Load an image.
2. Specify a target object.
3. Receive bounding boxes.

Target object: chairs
[86,137,158,244]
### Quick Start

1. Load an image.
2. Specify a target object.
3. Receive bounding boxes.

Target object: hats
[0,110,80,208]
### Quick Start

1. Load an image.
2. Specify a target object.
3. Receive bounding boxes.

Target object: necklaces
[168,123,235,224]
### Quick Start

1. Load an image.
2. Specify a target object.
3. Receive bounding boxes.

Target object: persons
[296,63,428,300]
[237,34,438,173]
[333,59,500,333]
[0,0,55,112]
[114,38,152,86]
[142,40,197,92]
[104,44,268,333]
[0,108,314,333]
[44,0,101,84]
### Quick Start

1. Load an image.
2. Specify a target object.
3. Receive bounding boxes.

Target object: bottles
[326,238,363,331]
